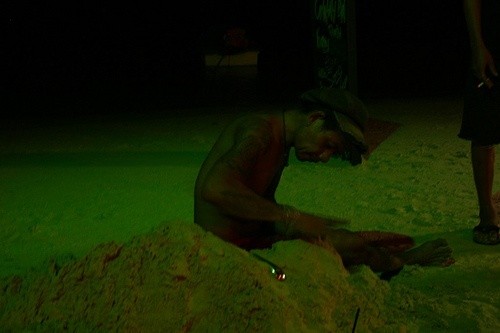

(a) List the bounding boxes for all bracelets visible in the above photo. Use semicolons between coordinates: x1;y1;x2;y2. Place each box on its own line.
274;202;303;238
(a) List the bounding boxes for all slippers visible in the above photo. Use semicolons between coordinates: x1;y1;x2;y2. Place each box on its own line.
472;224;500;245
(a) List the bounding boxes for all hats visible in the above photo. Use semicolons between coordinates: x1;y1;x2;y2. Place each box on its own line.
300;87;370;148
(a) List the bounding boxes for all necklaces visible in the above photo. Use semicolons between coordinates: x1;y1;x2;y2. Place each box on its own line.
279;107;293;170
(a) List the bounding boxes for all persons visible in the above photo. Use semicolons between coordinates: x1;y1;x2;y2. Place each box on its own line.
193;87;410;281
453;0;500;250
